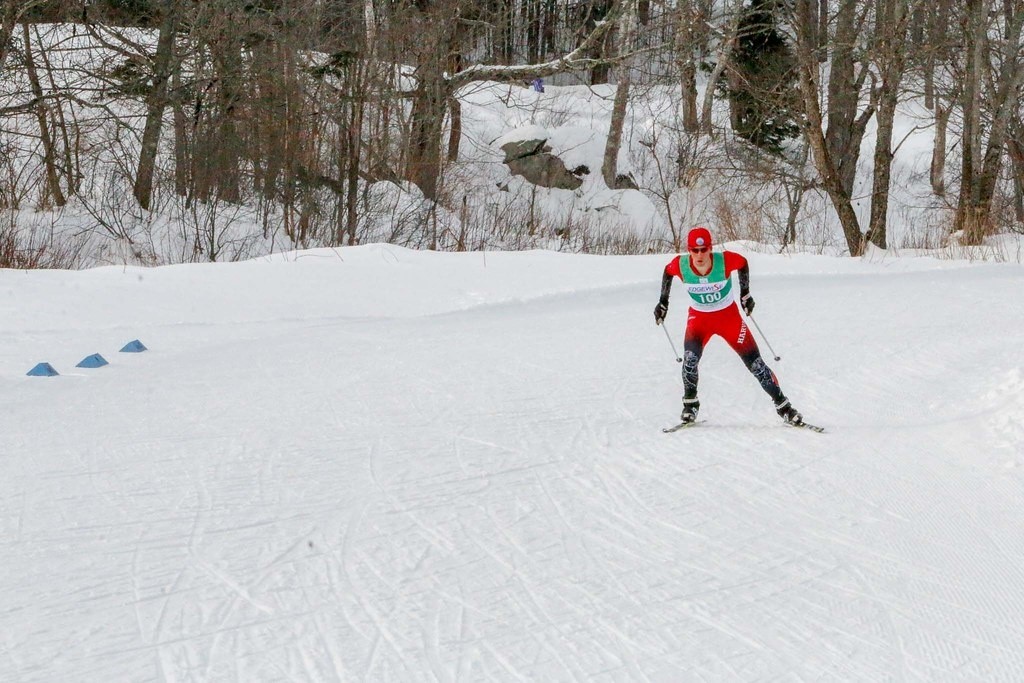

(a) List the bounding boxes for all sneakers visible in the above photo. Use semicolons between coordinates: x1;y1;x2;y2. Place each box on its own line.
775;398;803;425
681;397;700;422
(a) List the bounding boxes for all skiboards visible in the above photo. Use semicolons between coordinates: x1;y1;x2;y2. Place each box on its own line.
661;418;826;434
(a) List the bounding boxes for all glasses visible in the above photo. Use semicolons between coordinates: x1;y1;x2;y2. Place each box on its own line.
690;247;709;253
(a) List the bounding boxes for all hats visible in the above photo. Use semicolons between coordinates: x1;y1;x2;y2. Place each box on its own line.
687;228;712;253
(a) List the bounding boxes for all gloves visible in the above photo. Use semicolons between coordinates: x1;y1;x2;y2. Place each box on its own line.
654;298;668;325
740;288;755;316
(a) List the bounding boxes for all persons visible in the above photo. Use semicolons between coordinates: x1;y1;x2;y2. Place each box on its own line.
653;228;803;424
